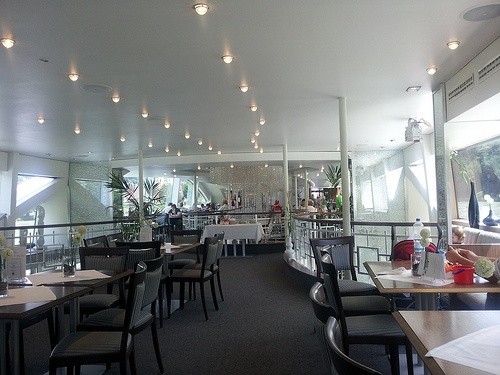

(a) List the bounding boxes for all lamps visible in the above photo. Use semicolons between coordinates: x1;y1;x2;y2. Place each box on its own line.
448;40;459;50
1;4;266;157
427;68;437;75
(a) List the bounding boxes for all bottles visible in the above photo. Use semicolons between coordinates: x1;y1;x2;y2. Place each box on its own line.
413;218;425;255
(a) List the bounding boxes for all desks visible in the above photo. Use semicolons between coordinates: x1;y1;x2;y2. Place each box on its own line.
142;242;201;316
363;261;500;375
391;310;500;375
204;222;262;259
0;284;88;375
9;267;135;375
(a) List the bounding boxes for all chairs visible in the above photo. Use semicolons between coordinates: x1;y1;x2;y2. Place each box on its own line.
263;208;338;239
49;214;224;375
308;235;413;375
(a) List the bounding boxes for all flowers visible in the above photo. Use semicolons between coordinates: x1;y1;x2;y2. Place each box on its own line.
449;144;493;211
419;228;431;251
68;226;86;266
0;234;13;269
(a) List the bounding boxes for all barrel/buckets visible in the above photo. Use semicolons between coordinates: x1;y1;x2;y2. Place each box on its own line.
451;265;474;284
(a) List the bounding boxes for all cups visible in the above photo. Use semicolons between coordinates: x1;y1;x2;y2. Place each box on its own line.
410;253;422;277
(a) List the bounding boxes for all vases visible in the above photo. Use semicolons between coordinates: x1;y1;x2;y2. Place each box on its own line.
62;258;75;277
417;250;427;274
0;269;10;298
468;180;498;229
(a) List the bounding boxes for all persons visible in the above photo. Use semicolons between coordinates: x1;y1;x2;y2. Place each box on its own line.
200;200;228;224
445;227;500;283
161;202;187;231
301;199;317;212
274;200;282;212
